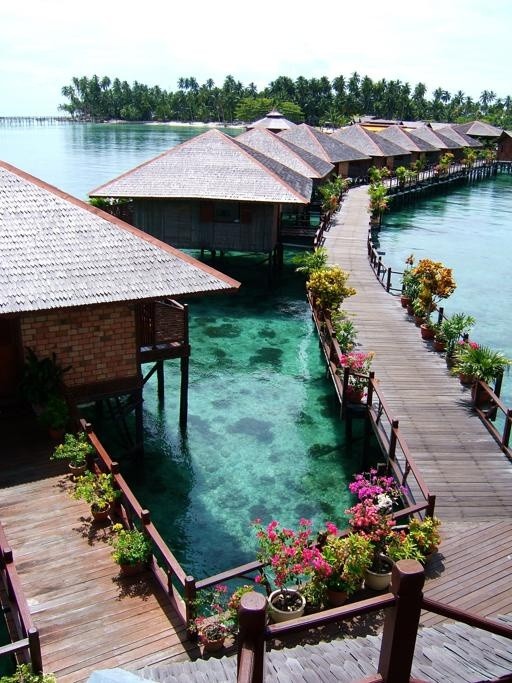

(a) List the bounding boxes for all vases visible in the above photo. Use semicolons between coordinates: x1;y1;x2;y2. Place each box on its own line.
366;553;394;591
267;587;307;621
200;638;226;654
419;547;438;571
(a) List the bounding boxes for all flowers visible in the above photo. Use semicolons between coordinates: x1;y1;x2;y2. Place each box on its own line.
407;515;444;553
346;500;401;570
186;582;257;642
250;515;332;608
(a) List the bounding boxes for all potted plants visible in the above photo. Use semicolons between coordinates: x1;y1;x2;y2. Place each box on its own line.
296;244;375;403
15;344;80;442
48;429;93;480
312;521;372;605
104;523;153;578
398;252;511;406
60;470;122;522
